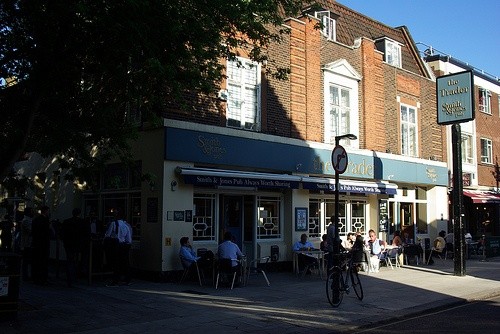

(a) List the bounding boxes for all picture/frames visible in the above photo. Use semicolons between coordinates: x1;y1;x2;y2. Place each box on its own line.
174;211;185;221
185;210;192;222
295;207;308;231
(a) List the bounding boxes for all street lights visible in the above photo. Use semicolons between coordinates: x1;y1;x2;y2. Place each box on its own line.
332;134;358;303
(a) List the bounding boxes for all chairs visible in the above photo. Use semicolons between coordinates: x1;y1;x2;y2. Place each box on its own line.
293;246;351;275
179;248;271;291
355;237;487;273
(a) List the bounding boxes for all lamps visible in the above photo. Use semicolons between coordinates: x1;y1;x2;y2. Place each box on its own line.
148;180;155;192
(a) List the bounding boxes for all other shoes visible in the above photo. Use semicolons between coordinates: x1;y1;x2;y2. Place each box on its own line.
105;283;119;288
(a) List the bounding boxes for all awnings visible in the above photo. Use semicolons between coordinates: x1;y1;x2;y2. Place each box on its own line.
293;174;398;194
174;167;301;189
463;191;500;203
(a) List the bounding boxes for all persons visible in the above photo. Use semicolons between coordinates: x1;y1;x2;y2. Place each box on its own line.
292;235;311;275
425;230;447;265
4;206;135;287
218;231;244;287
392;230;424;266
320;233;363;272
477;237;485;250
180;237;221;288
327;215;342;289
353;230;391;266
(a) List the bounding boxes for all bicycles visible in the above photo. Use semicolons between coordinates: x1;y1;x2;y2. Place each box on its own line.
325;251;369;308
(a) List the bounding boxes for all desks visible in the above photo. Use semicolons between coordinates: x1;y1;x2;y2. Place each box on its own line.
293;250;324;274
